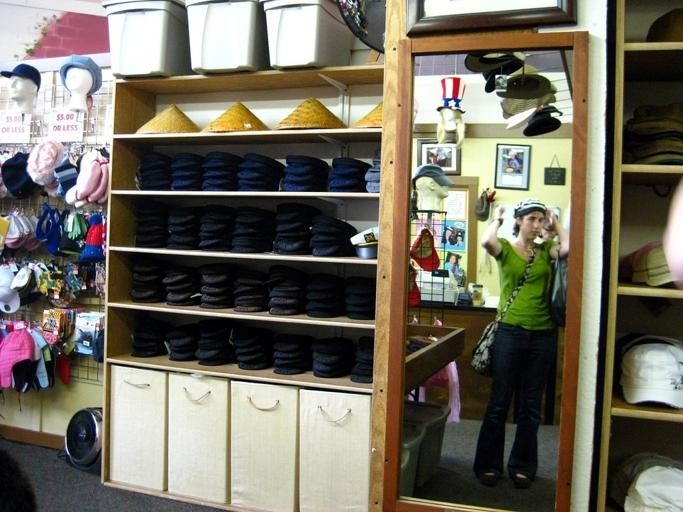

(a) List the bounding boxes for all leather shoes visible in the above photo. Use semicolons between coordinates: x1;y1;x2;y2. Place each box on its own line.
478;472;500;487
513;473;532;488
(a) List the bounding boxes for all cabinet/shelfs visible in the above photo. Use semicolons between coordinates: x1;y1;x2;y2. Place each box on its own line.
99;62;384;512
594;0;683;512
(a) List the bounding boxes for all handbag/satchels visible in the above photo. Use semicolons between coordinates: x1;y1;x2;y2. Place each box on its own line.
543;153;566;186
548;246;568;329
470;243;539;376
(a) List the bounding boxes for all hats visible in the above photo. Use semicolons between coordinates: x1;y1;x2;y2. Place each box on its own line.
198;263;233;309
622;104;682;165
201;151;241;190
351;335;374;383
202;102;272;131
436;78;466;114
1;322;56;393
60;54;102;96
309;215;358;257
306;272;345;318
167;207;201;250
620;332;683;411
233;271;268;312
513;200;547;218
273;202;322;255
647;8;683;42
506;108;537;130
233;327;272;370
84;156;109;209
131;201;169;248
475;190;490;222
624;466;683;512
131;320;168;357
2;152;35;200
26;141;64;185
163;265;201;306
500;93;556;119
262;265;304;315
281;155;331;191
237;152;286;191
507;65;538;80
65;154;101;205
631;240;680;287
231;206;274;253
0;265;20;314
345;276;376;320
412;164;455;191
409;228;440;272
135;104;199;134
36;203;71;258
169;153;204;191
1;64;41;88
364;149;381;192
350;101;383;127
464;50;517;73
273;334;309;375
409;264;420;306
312;337;354;378
199;204;232;251
482;64;525;93
330;157;372;192
191;319;233;366
276;98;347;129
130;260;164;303
606;452;682;507
495;75;551;99
166;323;199;362
75;150;108;208
524;112;561;136
80;214;105;262
136;152;173;190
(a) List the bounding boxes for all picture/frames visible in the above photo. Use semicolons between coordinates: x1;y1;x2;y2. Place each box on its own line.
495;143;532;191
416;138;461;176
400;0;578;35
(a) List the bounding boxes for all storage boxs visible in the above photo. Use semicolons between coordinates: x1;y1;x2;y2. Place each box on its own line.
102;0;184;77
258;0;353;69
183;0;261;72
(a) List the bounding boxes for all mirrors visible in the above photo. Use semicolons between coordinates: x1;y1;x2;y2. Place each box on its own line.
385;32;593;512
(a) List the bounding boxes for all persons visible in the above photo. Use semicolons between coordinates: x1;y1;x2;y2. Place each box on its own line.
444;252;459;275
443;225;465;248
0;64;41;113
413;164;454;211
473;200;565;487
427;151;437;162
507;150;522;174
436;76;466;147
61;54;102;109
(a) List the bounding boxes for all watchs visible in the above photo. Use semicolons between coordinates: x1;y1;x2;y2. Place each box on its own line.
492;218;503;224
